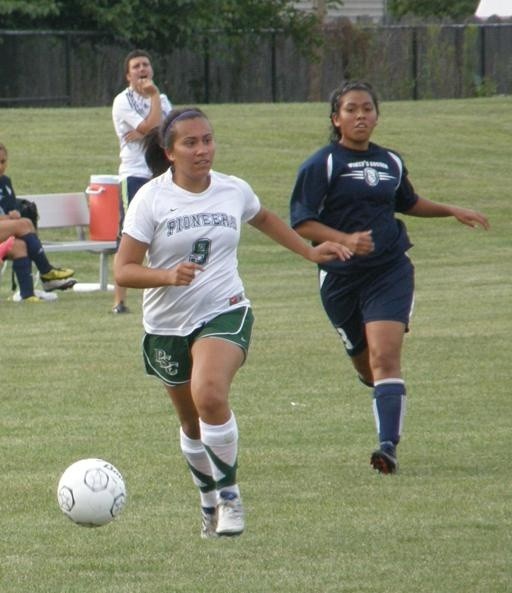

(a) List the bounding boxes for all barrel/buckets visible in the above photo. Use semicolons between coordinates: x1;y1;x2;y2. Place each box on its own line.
86;173;121;242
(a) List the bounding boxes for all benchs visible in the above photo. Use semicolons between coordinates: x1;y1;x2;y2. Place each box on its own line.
0;192;118;297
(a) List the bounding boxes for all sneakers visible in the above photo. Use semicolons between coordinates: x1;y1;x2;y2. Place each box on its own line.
21;295;49;303
201;507;217;539
40;266;74;282
42;278;77;292
370;446;398;474
216;485;243;536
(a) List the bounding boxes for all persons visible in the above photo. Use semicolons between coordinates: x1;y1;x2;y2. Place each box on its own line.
112;101;356;541
0;140;78;301
109;47;174;315
287;76;492;477
0;209;78;304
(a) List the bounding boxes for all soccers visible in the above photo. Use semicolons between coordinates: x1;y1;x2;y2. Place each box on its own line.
57;457;127;528
